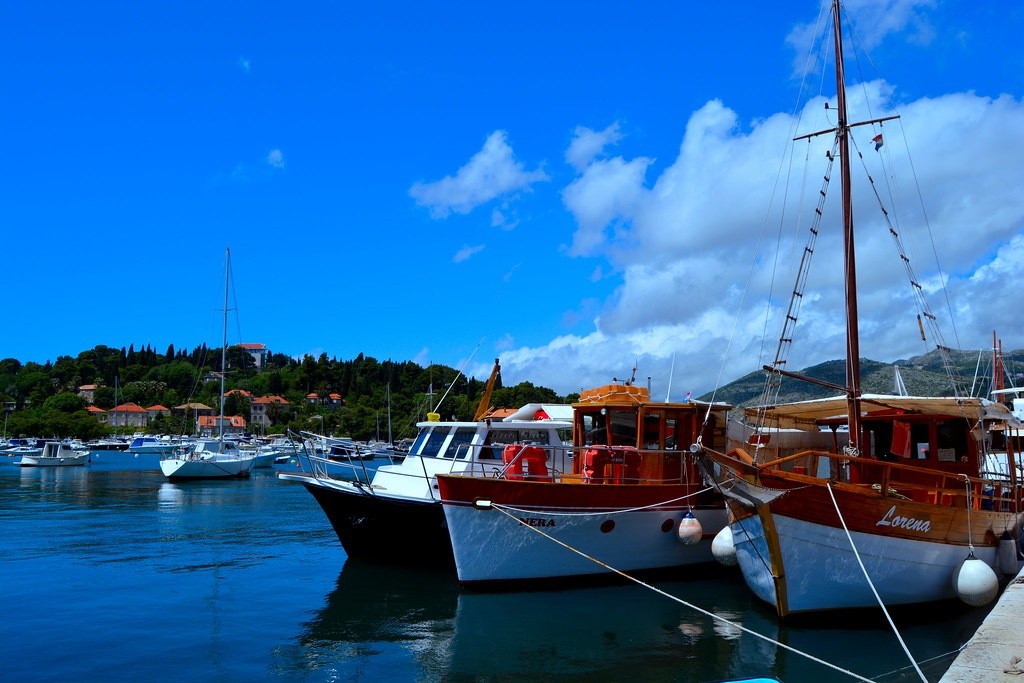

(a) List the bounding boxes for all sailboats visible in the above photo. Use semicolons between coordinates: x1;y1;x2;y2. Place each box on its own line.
1;2;1024;614
